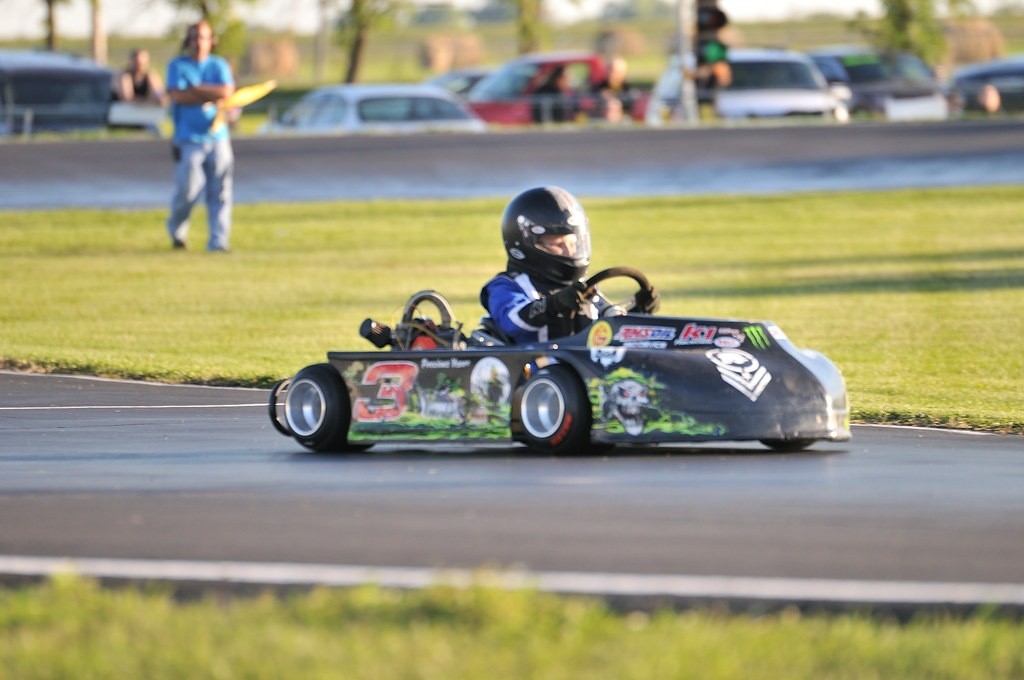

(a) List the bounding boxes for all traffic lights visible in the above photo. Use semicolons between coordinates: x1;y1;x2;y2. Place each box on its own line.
693;0;729;100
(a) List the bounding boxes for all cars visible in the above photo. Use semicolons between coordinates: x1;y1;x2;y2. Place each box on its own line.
433;49;658;129
948;55;1023;117
646;50;854;125
259;81;489;135
814;48;947;125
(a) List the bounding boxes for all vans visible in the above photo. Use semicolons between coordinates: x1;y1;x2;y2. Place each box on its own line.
0;51;118;137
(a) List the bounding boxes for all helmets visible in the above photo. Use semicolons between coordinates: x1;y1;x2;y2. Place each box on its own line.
500;185;591;282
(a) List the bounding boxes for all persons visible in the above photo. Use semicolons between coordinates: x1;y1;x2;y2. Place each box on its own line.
103;48;163;130
164;18;241;252
529;64;588;126
592;58;642;121
963;83;1007;118
478;185;659;344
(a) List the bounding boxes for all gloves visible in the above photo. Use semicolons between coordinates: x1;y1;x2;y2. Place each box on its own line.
547;281;588;312
634;285;661;315
